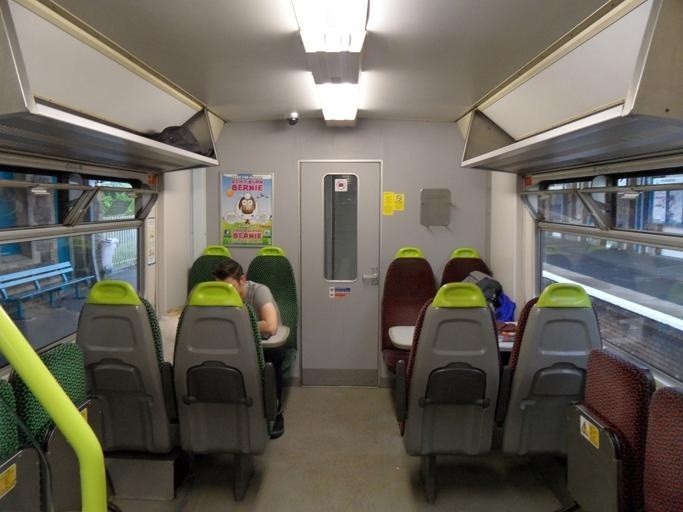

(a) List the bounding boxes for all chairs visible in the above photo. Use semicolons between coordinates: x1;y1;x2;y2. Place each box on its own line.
245;246;298;371
404;283;500;505
172;282;277;502
439;248;491;288
187;245;233;298
643;386;683;512
558;348;656;512
495;283;602;454
75;280;172;454
381;248;436;375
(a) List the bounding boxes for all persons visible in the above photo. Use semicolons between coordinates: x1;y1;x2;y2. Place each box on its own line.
214;258;285;440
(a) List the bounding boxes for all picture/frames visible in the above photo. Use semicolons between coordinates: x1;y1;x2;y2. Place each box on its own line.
219;172;275;248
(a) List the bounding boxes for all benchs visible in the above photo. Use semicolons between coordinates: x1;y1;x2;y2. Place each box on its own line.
0;261;96;319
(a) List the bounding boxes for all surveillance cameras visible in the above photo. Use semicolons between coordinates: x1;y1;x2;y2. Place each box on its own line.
287;112;299;125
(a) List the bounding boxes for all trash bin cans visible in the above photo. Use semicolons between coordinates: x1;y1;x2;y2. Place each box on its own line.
98;237;119;274
